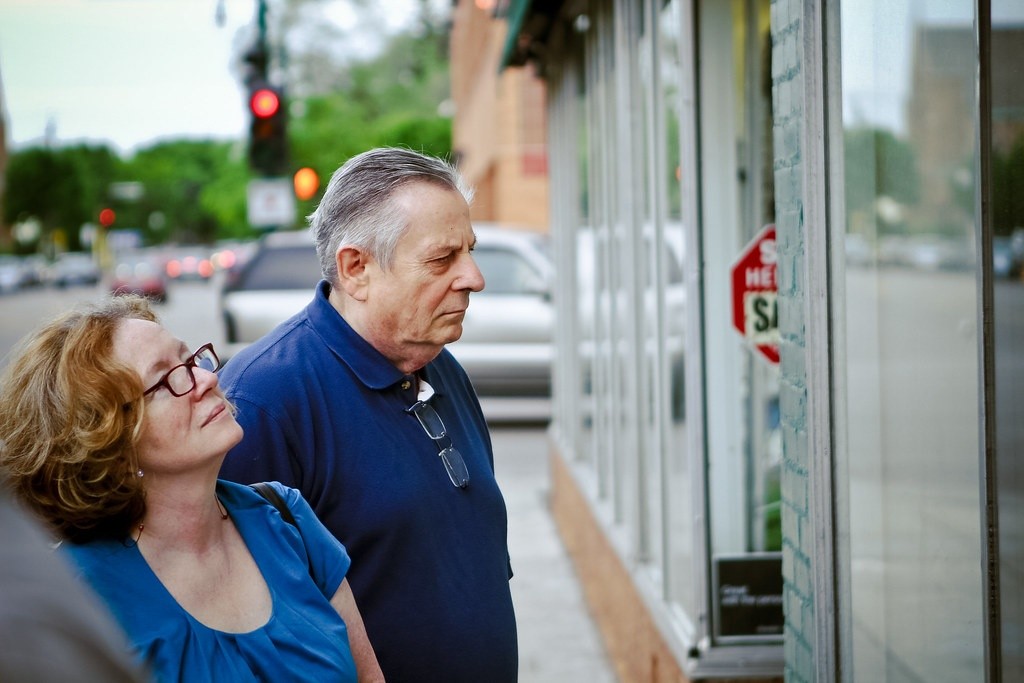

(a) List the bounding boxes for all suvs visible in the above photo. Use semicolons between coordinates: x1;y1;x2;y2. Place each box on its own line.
578;225;686;405
219;225;556;408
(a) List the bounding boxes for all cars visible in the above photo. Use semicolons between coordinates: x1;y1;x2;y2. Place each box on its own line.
112;261;169;304
844;230;1022;278
1;253;95;292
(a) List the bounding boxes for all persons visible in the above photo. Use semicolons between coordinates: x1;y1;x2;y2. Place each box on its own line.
216;146;519;683
0;293;388;683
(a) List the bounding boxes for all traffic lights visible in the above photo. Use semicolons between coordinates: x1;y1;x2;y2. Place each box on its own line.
248;85;287;175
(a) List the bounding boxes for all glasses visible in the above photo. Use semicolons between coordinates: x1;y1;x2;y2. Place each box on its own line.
413;400;470;489
121;341;221;409
